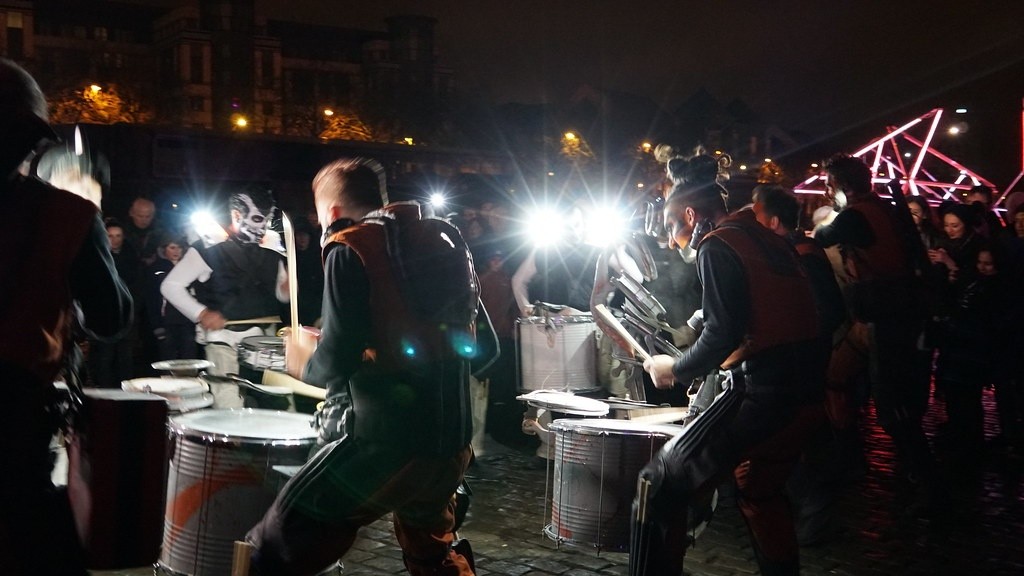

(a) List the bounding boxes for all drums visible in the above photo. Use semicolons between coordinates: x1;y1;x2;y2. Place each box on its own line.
155;405;343;576
237;331;322;413
57;386;169;573
121;376;217;461
546;409;690;553
515;313;602;395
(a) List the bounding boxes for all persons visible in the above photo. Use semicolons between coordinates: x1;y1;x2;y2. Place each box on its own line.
511;139;1023;576
0;59;520;576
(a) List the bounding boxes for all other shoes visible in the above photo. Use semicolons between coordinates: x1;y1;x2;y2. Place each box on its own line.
796;507;847;545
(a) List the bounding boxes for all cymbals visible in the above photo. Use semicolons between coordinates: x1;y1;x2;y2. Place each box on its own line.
149;358;217;374
597;394;659;407
516;390;609;417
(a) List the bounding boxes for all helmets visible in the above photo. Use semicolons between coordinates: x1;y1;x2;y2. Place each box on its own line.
0;57;62;143
